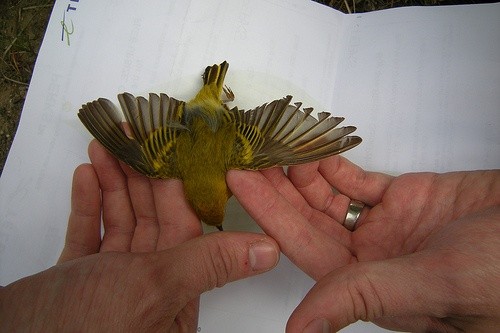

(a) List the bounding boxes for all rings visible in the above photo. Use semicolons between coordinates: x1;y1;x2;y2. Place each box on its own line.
344;197;365;230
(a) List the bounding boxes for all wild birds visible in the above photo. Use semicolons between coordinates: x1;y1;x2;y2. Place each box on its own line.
77;60;363;231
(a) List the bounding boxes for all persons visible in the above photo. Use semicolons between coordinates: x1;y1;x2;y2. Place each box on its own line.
0;120;500;333
